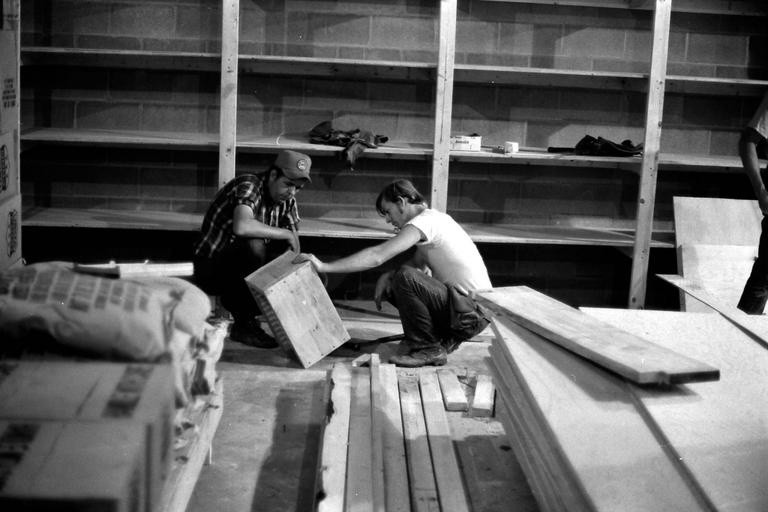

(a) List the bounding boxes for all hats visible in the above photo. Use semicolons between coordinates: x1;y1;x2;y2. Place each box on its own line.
271;148;315;184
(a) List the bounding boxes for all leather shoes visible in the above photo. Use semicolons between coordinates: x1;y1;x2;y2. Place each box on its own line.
387;341;449;368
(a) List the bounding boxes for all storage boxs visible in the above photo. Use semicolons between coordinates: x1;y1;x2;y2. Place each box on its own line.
0;418;152;511
0;361;177;511
249;250;348;369
1;0;27;271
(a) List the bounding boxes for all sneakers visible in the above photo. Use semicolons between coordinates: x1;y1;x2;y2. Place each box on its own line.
226;318;280;351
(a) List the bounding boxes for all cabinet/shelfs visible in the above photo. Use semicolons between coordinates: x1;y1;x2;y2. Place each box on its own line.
20;0;766;347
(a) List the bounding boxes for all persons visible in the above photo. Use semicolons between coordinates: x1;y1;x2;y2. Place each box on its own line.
292;175;495;368
737;93;767;318
186;150;314;349
